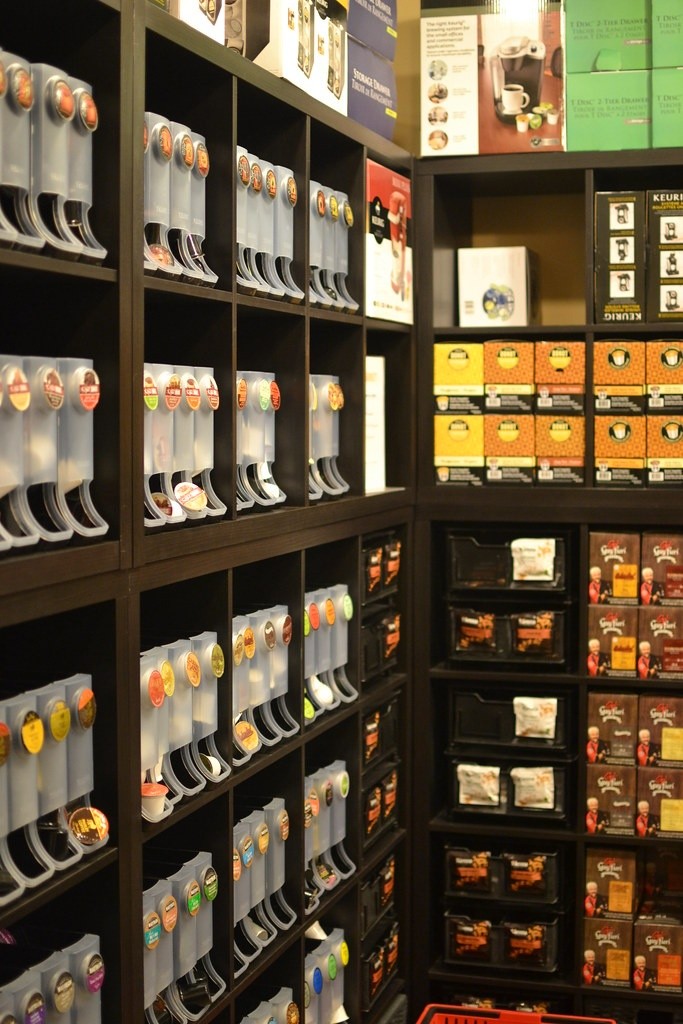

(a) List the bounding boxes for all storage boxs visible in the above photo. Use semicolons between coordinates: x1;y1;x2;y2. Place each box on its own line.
366;160;683;327
361;529;683;1023
147;0;683;158
365;336;683;493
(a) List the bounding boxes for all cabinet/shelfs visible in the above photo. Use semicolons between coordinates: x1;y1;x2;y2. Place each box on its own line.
133;0;417;568
415;147;683;1023
1;570;132;1024
0;0;133;595
133;506;414;1024
